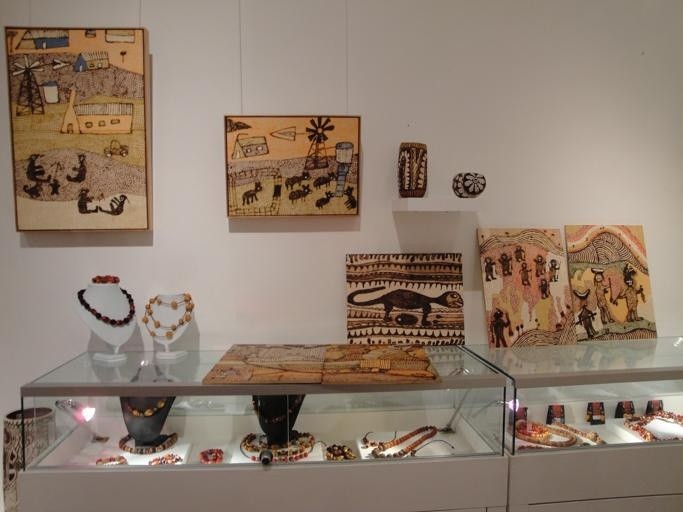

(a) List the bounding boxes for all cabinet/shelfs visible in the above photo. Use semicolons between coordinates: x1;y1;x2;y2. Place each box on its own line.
465;336;681;511
14;335;509;512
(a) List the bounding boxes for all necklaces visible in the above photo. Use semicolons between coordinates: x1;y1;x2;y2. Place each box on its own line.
510;410;683;450
77;275;194;339
252;395;302;424
126;397;168;417
